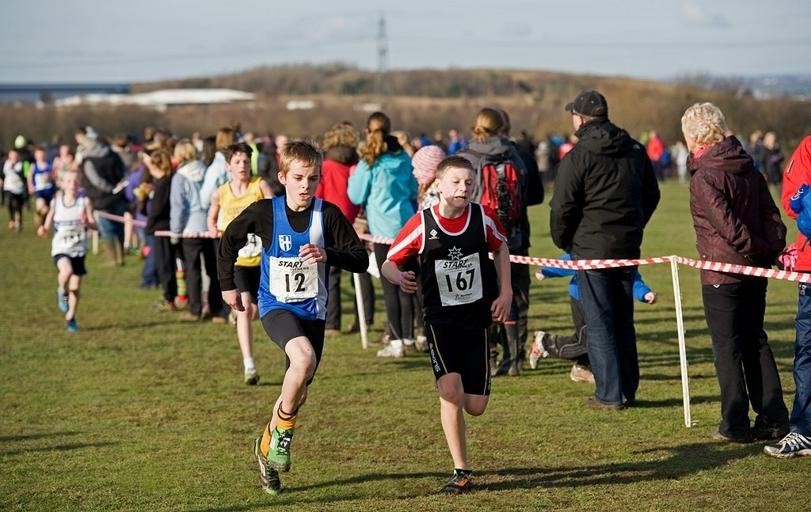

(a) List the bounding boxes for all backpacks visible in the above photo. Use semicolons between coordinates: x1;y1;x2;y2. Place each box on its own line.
463;142;521;236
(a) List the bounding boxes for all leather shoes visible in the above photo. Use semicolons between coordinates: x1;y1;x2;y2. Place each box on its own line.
587;396;624;411
710;428;754;442
622;393;636;406
751;422;790;440
178;311;200;322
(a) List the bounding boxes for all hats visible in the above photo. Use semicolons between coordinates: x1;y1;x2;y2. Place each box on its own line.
411;145;447;184
565;89;608;118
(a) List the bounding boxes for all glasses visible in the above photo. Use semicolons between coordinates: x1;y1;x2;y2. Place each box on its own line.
570;108;577;116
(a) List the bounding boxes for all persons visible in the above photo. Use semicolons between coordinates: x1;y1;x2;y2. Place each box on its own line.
26;145;54;217
682;103;789;440
199;126;238;212
409;146;445;211
73;125;96;157
549;89;662;409
208;145;275;384
143;149;181;309
390;128;430;158
173;139;222;323
316;121;375;335
114;151;151;252
126;127;173;151
457;109;532;377
39;161;100;331
432;129;466;153
193;132;213;164
499;109;542;372
347;112;420;357
50;144;72;172
747;128;786;183
382;156;512;494
216;141;370;493
243;131;289;194
532;128;580;172
5;149;28;232
76;137;127;264
530;251;656;383
644;130;690;183
764;131;811;456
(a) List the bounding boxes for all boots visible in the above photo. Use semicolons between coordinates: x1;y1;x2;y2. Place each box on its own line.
489;350;501;377
504;320;518;376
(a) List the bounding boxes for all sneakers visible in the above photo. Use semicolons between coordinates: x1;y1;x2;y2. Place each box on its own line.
401;338;418;354
377;339;406;358
380;334;391;344
174;296;188;311
267;424;294;473
415;335;429;352
65;314;78;333
8;221;14;229
155;300;176;313
343;324;371;335
570;363;596;383
56;287;69;313
441;469;473;494
252;434;282;496
243;358;260;385
324;327;343;339
763;431;811;457
529;330;550;370
14;223;21;233
